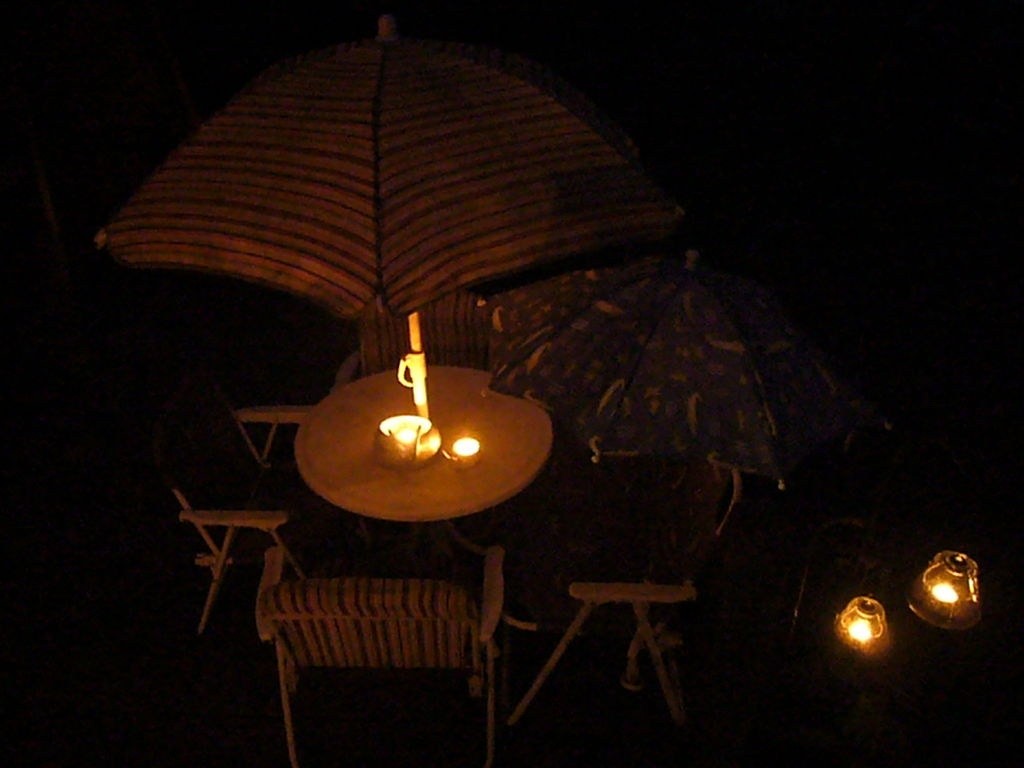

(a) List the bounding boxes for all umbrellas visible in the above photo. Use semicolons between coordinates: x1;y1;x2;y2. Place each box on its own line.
467;249;867;491
89;13;689;323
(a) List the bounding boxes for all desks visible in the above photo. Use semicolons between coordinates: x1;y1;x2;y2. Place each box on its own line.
292;365;553;522
779;514;933;737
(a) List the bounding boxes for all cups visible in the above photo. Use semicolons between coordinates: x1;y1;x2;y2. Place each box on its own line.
451;437;481;467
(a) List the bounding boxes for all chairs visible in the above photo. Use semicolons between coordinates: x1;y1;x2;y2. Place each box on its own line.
499;457;745;725
256;544;506;768
147;369;308;633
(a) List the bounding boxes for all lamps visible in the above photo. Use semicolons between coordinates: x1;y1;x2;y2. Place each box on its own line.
832;597;890;697
905;550;982;630
